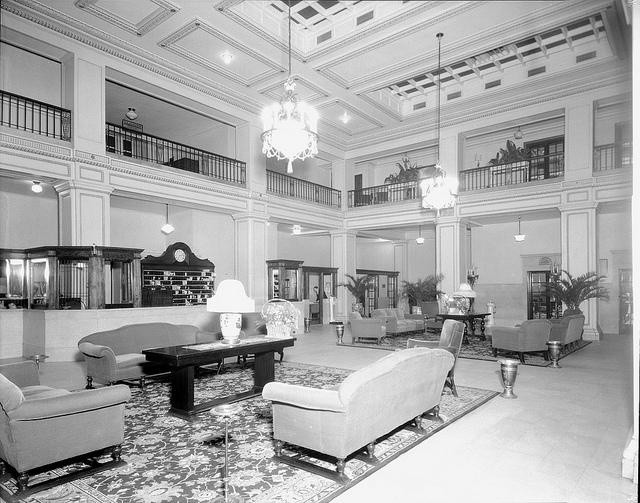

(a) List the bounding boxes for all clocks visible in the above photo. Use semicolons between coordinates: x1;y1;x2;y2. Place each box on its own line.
174;248;186;263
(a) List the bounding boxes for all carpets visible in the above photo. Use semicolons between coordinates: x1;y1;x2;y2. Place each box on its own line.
0;359;500;503
344;327;594;369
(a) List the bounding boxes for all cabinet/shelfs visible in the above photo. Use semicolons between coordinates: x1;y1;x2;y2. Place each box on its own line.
306;299;321;320
143;265;215;308
267;266;303;302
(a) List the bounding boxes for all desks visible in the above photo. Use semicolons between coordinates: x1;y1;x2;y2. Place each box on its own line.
136;331;301;421
434;307;492;344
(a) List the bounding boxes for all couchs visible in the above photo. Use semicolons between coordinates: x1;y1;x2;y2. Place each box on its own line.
0;352;134;503
347;302;430;345
76;322;203;391
258;339;455;483
485;312;587;367
402;317;466;399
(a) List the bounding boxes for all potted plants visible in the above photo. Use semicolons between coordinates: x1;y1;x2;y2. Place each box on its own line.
397;273;447;314
335;272;377;313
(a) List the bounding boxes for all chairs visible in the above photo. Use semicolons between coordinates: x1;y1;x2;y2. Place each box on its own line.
262;295;300;337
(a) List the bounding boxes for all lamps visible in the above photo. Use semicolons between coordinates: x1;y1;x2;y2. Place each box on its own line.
205;277;257;344
158;203;175;235
28;178;44;194
420;30;461;219
416;224;424;244
515;126;524;139
261;0;321;174
453;282;476;312
218;49;235;64
289;222;304;235
125;105;139;119
513;215;526;241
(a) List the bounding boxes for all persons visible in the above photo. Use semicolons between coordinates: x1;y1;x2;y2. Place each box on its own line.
313;285;327;301
